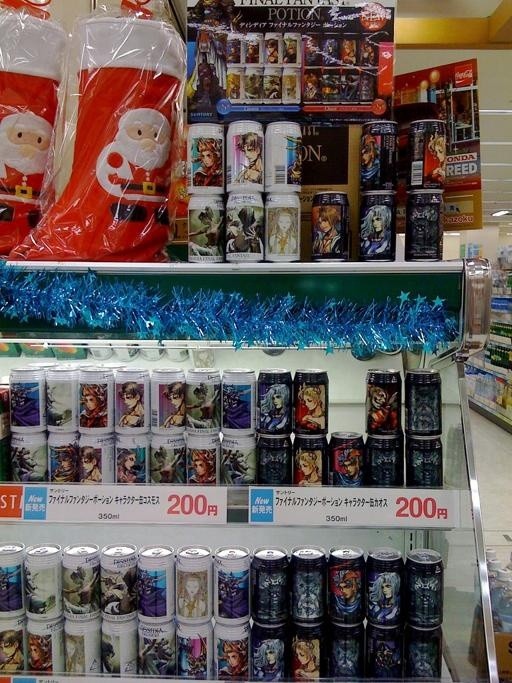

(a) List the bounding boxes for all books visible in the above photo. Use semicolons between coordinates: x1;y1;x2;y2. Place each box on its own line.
463;270;510;426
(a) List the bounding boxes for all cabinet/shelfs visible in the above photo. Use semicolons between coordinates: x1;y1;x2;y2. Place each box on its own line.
1;255;500;682
463;269;512;436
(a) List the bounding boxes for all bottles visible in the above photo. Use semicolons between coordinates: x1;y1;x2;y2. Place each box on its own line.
463;362;495;404
484;296;512;369
475;547;512;632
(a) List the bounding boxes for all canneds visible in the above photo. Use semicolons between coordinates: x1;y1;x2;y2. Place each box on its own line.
402;623;442;683
327;432;364;487
219;434;256;487
292;369;329;438
150;366;186;435
255;431;293;487
287;623;326;683
27;361;126;368
137;620;176;679
408;119;448;193
26;617;65;676
225;31;378;105
405;435;443;489
25;543;64;622
9;367;47;434
403;549;444;630
365;368;402;435
113;367;151;435
221;368;255;436
256;368;294;436
64;615;101;677
250;547;290;626
136;545;176;625
46;431;81;483
78;434;114;486
186;120;302;263
365;547;405;629
10;432;48;483
185;368;221;438
328;624;366;683
100;617;138;678
0;614;27;675
290;545;328;628
174;544;214;625
61;543;102;623
100;543;138;623
361;194;399;263
114;432;151;486
364;625;406;683
45;366;79;433
359;119;399;193
186;435;221;487
213;621;249;682
406;189;444;263
310;193;349;261
404;367;442;437
0;542;27;619
249;621;290;682
78;366;116;435
364;434;405;487
213;545;252;625
175;619;214;680
327;545;366;627
291;434;329;487
149;432;187;487
